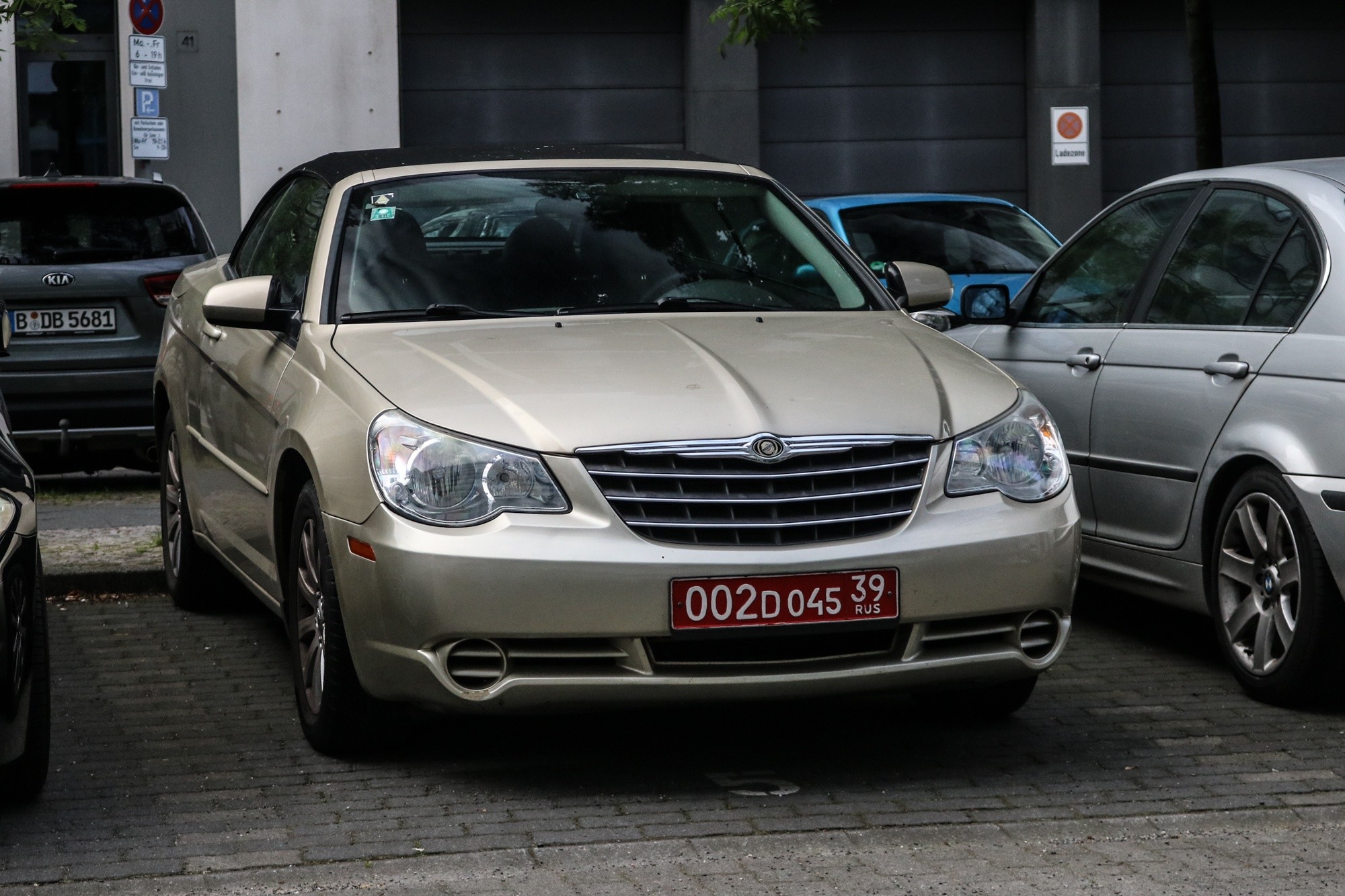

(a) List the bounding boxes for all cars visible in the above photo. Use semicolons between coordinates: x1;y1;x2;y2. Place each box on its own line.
726;192;1100;332
933;157;1345;701
0;418;53;799
150;149;1080;750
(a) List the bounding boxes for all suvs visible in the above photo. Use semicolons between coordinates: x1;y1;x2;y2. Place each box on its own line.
0;173;217;474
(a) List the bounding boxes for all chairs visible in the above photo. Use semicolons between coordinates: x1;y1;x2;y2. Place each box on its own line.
583;199;685;306
343;205;439;319
